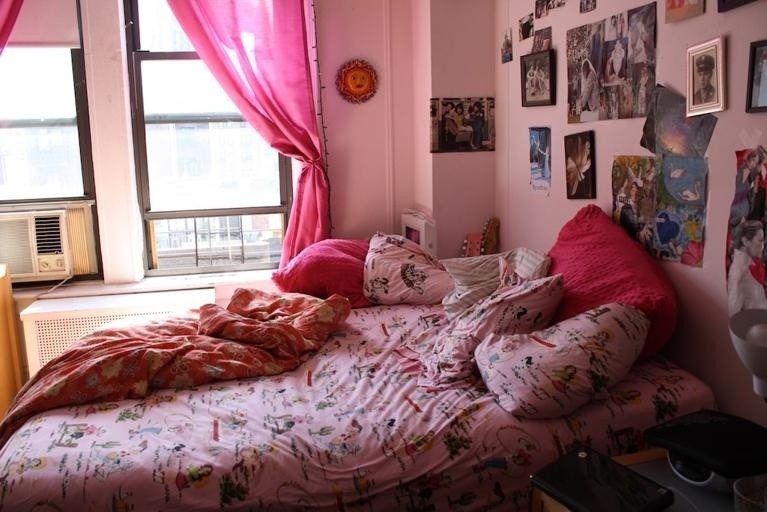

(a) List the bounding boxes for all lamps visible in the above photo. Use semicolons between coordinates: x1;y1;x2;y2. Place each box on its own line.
728;308;766;404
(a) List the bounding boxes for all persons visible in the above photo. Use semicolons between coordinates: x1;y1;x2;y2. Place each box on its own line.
530;134;540;162
502;1;593;63
440;102;484;149
567;137;591;196
694;56;716;104
754;54;766;106
527;61;549;94
727;146;767;312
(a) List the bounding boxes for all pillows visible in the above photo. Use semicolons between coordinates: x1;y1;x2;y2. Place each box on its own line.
271;238;377;310
547;205;676;352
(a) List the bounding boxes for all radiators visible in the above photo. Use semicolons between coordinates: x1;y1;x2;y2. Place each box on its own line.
21;287;218;382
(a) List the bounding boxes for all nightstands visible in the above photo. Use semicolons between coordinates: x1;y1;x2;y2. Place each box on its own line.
531;406;767;510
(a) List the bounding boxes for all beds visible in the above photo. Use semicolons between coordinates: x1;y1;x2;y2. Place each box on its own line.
0;251;721;512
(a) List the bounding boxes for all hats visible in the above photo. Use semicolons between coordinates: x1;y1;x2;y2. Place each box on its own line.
696;55;715;72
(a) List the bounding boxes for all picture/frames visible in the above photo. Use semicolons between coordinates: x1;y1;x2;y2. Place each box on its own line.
744;39;767;113
685;35;728;118
563;129;596;199
519;49;556;108
